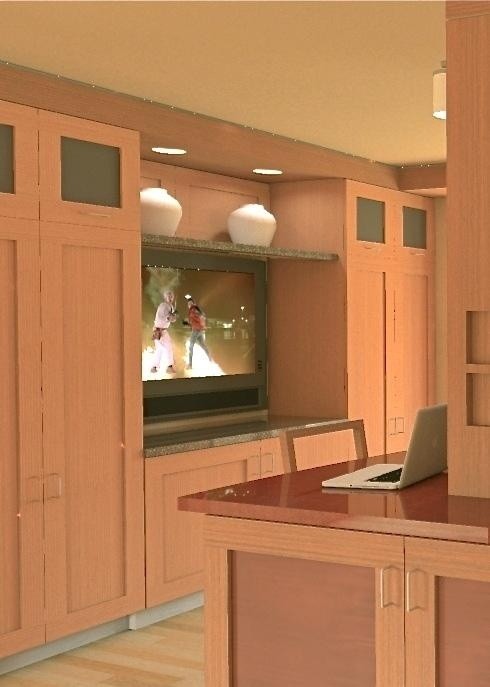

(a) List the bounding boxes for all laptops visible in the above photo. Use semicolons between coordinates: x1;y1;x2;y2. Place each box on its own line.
322;403;447;490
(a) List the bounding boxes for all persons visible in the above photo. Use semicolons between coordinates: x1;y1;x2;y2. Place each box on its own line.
149;291;178;373
182;299;213;370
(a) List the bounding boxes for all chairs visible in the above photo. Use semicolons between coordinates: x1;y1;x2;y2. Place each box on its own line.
277;418;368;472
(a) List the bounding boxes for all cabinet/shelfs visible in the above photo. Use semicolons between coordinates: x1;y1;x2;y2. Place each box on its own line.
2;217;145;663
145;427;349;608
268;257;433;459
1;98;144;231
201;513;490;687
271;177;437;257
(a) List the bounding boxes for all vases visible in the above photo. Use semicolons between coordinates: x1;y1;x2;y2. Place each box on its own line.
226;201;279;247
140;186;183;236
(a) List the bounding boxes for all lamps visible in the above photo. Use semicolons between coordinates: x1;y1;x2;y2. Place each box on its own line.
432;61;447;120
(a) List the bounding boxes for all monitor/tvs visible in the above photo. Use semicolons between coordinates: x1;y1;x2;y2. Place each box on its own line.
141;248;268;398
